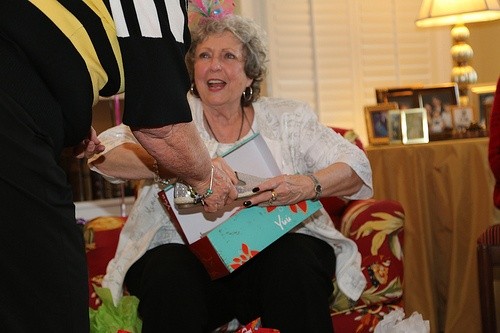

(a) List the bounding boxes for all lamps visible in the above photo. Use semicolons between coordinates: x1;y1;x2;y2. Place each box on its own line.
414;0;500;109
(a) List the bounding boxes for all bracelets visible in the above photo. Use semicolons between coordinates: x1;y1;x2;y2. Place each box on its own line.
189;164;213;205
152;158;162;182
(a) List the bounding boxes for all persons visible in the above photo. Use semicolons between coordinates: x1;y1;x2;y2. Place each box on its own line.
0;0;239;333
378;94;493;140
90;17;374;333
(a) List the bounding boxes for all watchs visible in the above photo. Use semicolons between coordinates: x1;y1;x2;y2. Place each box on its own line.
306;174;322;202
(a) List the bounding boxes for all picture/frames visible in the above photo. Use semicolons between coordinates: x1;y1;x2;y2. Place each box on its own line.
364;82;497;145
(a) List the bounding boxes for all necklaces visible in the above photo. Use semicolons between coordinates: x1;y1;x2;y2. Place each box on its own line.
204;105;247;143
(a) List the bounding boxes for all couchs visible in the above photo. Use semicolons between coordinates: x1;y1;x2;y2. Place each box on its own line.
86;127;407;333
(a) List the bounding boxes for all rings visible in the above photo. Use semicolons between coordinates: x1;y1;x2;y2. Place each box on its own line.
269;200;272;206
271;190;279;200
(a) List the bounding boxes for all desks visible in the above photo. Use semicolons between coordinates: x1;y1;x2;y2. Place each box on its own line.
360;137;500;333
